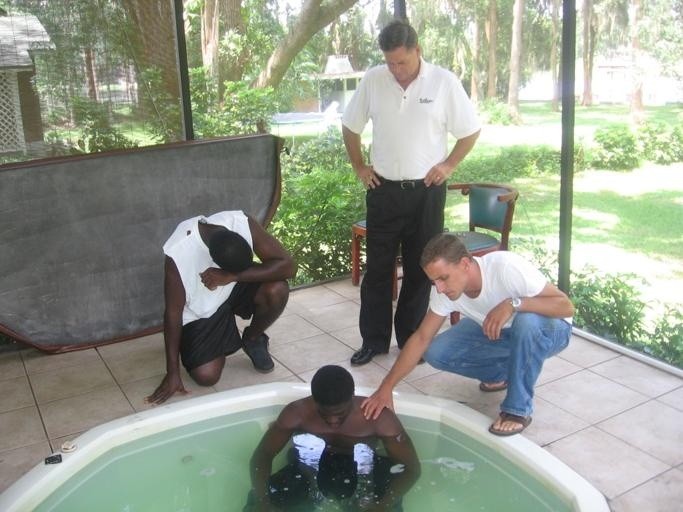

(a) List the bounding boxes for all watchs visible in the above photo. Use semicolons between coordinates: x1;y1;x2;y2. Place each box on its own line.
511;296;522;312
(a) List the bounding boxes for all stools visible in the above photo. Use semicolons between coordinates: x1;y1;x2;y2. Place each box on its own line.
351;219;404;300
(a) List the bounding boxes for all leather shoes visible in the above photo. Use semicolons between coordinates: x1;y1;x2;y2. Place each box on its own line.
350;345;382;366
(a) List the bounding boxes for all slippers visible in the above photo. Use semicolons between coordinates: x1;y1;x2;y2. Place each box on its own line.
489;413;532;436
480;379;508;392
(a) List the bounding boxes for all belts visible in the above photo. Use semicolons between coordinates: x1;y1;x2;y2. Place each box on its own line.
376;180;426;190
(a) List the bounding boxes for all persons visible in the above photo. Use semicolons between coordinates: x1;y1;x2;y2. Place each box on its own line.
243;365;421;512
361;234;574;436
340;22;483;367
144;210;297;407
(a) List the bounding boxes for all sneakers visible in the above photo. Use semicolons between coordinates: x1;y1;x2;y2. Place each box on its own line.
242;326;275;374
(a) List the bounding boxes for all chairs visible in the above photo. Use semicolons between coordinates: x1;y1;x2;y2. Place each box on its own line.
448;183;518;328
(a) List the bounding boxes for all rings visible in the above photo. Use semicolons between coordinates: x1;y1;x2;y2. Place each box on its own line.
437;176;440;180
